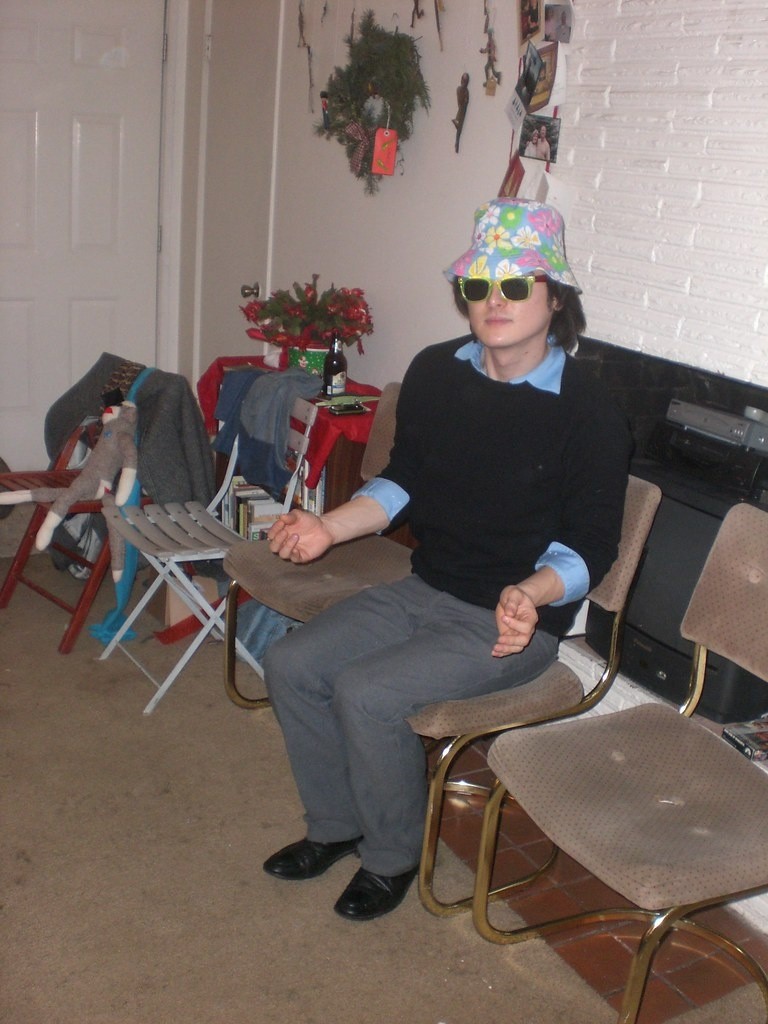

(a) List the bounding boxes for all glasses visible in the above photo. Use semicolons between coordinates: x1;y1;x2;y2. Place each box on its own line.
457;274;548;303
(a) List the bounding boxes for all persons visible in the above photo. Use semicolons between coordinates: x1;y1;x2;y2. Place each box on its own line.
521;0;538;36
263;197;631;921
524;126;550;160
545;7;570;43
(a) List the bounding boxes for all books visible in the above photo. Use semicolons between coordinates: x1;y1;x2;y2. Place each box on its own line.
222;460;323;540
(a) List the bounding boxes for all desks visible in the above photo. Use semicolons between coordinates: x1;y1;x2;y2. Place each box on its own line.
196;355;382;521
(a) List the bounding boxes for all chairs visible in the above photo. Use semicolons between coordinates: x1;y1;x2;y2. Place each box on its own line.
219;383;413;710
409;475;662;920
100;395;318;719
475;504;768;1024
0;350;197;655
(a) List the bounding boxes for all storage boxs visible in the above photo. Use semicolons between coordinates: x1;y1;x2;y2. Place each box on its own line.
146;563;219;628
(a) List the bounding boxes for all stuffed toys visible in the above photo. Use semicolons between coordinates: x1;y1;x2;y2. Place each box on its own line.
0;401;138;584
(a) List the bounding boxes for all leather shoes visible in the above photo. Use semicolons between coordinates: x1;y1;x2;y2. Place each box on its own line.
334;863;422;921
263;834;364;881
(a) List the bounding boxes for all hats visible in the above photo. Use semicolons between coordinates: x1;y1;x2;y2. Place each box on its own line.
443;197;582;294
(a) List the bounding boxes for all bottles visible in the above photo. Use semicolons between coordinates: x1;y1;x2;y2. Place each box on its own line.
323;329;346;397
